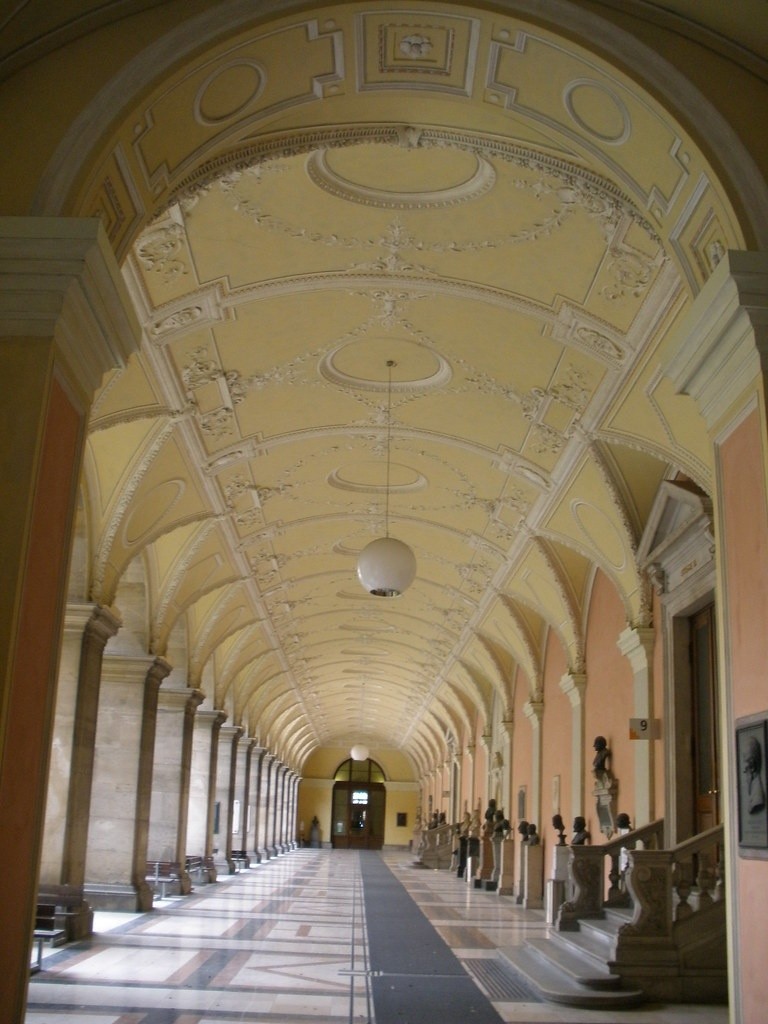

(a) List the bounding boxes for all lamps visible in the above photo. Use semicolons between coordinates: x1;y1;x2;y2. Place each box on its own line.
356;359;417;596
351;684;370;761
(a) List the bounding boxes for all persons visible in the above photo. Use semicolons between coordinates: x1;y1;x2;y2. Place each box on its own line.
571;816;591;844
552;815;565;831
592;736;611;781
459;798;512;838
517;820;539;845
428;813;446;829
616;813;635;849
